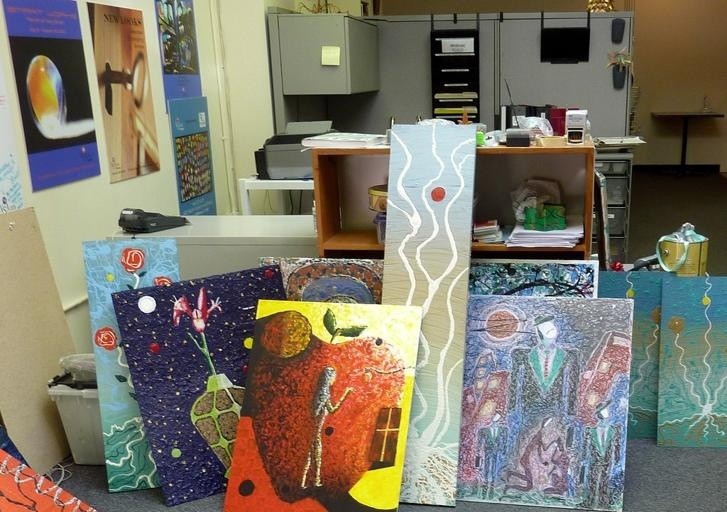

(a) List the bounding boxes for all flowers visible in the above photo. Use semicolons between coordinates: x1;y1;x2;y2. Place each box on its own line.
166;286;226;375
603;45;633;73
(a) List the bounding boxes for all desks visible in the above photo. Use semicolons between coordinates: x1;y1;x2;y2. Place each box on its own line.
650;112;724;163
237;177;315;215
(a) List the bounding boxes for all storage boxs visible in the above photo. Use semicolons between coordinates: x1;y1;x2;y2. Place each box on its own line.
373;213;386;245
48;384;109;468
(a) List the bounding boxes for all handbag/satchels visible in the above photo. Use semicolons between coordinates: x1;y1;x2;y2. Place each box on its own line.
512;189;550;223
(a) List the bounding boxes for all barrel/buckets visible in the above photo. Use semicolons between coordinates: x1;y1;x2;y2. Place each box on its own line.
657;233;708;278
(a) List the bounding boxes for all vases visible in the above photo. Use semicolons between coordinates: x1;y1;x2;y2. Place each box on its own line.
612;65;626;89
190;372;246;477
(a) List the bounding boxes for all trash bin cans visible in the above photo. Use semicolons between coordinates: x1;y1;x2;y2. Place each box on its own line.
47;354;104;465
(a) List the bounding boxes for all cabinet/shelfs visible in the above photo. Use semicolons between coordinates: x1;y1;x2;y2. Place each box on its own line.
591;148;634;264
102;211;320;280
312;134;596;260
276;11;379;97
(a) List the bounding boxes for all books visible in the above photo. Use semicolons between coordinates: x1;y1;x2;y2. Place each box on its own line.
594;134;648;147
301;132;384;147
472;217;582;250
